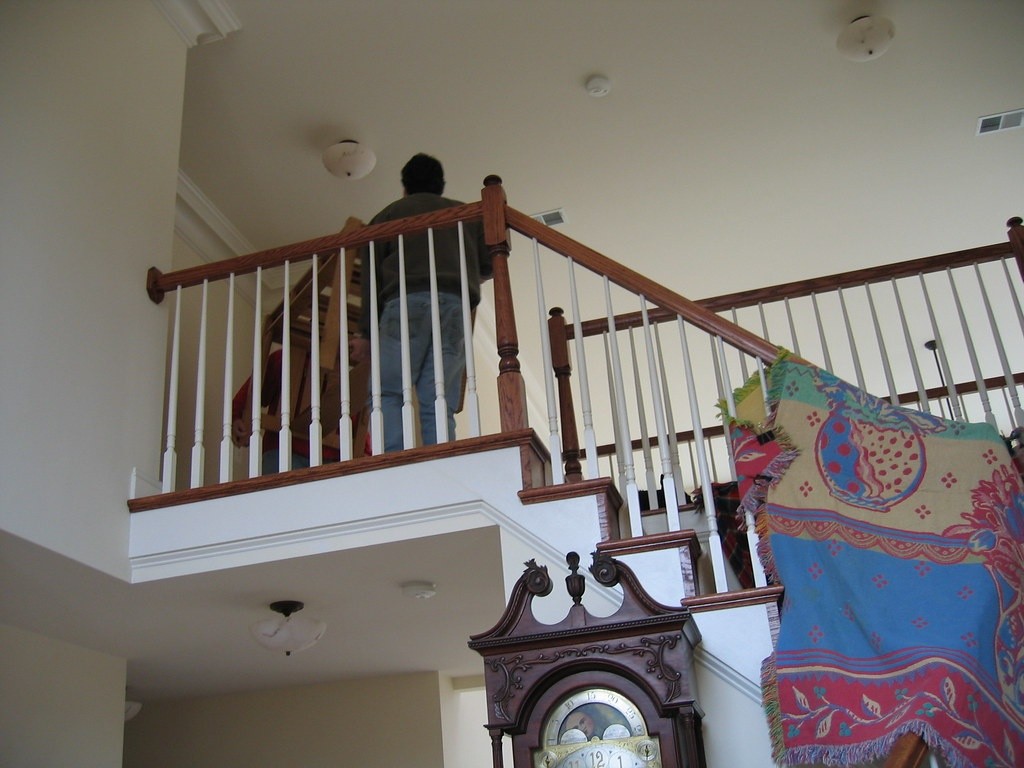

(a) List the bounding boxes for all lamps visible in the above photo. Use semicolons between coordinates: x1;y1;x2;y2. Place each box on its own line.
248;600;327;657
835;14;897;62
322;139;376;180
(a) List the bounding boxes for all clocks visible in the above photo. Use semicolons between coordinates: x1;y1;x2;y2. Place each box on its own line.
466;551;707;768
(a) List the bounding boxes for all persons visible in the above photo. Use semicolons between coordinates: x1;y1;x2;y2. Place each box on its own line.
356;153;493;454
231;350;374;477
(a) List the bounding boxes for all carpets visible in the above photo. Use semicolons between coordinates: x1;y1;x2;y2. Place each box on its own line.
715;347;1024;768
